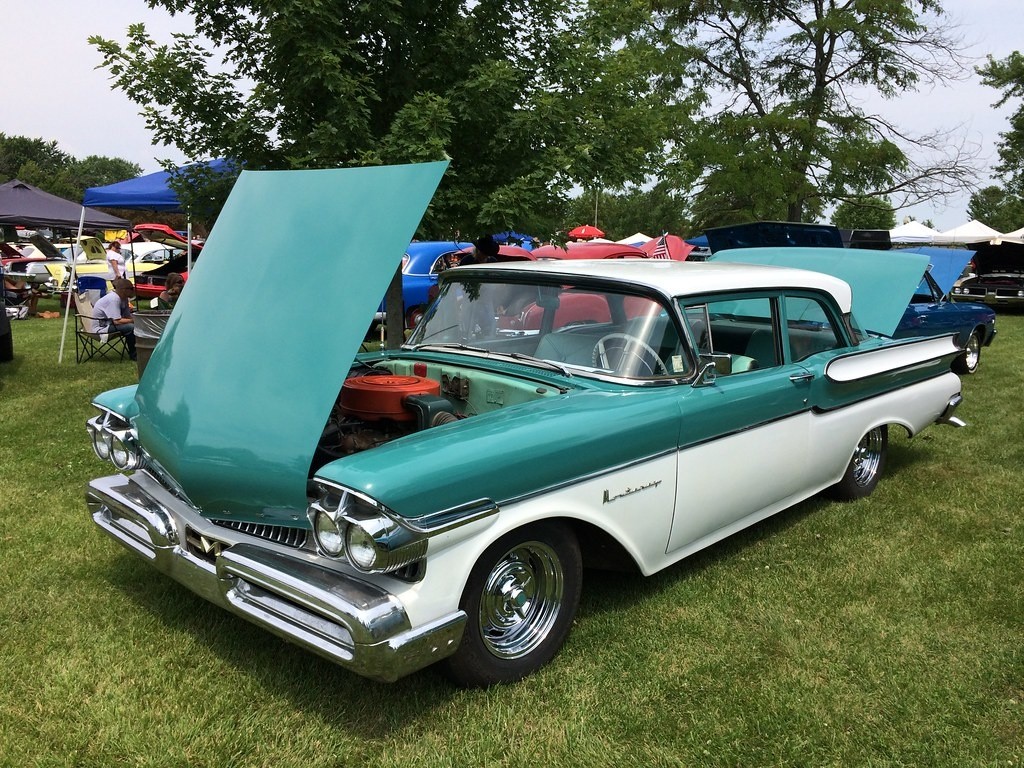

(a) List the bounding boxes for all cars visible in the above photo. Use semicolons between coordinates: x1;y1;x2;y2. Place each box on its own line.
372;241;475;332
84;158;963;688
452;235;701;332
893;246;997;375
132;222;203;297
0;234;205;292
949;242;1024;315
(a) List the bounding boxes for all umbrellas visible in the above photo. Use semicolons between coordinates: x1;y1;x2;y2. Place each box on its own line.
568;225;605;237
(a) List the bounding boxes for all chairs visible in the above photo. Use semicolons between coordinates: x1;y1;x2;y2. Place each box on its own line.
72;277;132;362
664;348;757;377
4;286;33;307
534;333;600;366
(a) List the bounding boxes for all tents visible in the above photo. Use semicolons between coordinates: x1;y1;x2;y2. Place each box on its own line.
0;178;131;231
83;156;251;212
889;219;1024;244
588;231;711;246
494;231;539;246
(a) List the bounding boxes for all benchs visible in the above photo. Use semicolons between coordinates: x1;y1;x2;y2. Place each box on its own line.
629;318;840;369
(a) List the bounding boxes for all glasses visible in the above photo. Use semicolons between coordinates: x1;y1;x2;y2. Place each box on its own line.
115;247;122;250
175;280;185;284
122;287;135;290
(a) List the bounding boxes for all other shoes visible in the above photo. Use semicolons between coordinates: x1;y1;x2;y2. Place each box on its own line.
27;312;37;317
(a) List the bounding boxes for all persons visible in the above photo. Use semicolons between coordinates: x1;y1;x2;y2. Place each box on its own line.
90;279;138;362
106;241;128;289
159;272;185;308
0;254;53;299
